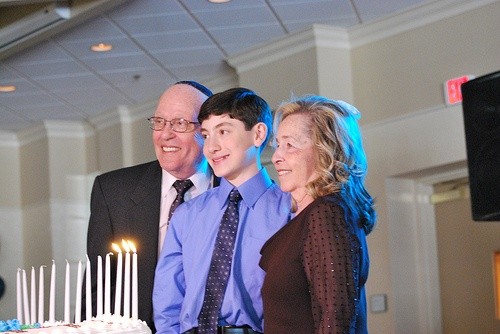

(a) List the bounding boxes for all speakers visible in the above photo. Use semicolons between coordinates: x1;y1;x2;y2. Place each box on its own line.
460;70;500;222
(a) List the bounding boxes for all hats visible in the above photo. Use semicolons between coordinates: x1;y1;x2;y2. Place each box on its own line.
175;81;214;98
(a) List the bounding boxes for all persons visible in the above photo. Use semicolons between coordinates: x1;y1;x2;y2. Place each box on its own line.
152;87;295;334
73;80;220;334
0;276;6;301
257;95;378;334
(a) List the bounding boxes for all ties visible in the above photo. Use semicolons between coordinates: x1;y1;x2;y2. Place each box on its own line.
197;190;243;334
165;178;195;232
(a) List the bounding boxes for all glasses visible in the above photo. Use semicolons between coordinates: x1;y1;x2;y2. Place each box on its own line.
147;116;201;134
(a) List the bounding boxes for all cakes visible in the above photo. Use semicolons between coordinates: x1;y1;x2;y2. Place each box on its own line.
0;313;152;334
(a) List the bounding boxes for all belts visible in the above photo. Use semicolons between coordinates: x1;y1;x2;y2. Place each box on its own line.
182;325;259;334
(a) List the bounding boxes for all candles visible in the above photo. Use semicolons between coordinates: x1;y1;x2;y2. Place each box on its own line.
15;267;22;326
122;239;130;318
131;242;139;319
65;259;71;323
38;264;47;324
30;265;36;323
97;254;102;315
85;252;92;319
48;258;55;322
113;242;122;317
22;269;29;324
104;253;113;315
74;259;82;323
